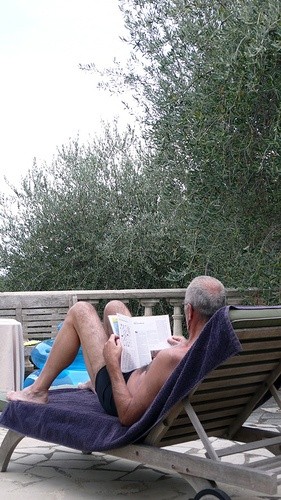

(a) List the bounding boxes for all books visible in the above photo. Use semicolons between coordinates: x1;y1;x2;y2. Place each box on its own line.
108;312;173;374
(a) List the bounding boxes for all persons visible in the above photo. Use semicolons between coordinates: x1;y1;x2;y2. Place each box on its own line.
8;274;229;426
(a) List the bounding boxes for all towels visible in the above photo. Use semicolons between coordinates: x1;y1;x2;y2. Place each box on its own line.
1;305;281;453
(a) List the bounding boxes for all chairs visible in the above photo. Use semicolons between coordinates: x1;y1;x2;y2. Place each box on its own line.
0;306;281;500
